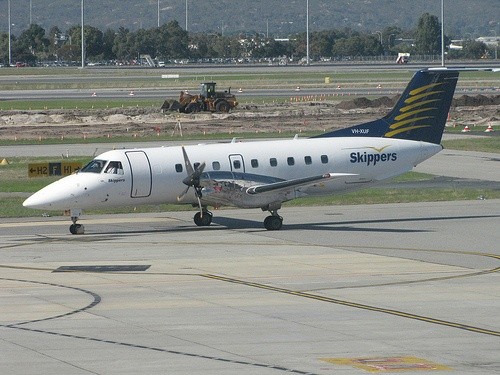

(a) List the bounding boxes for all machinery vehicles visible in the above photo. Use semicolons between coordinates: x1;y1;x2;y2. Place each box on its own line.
162;79;239;114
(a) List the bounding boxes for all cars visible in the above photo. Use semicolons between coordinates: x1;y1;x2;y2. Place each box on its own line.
1;57;167;67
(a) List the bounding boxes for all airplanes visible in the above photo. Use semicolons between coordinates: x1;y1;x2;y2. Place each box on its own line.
21;68;461;232
